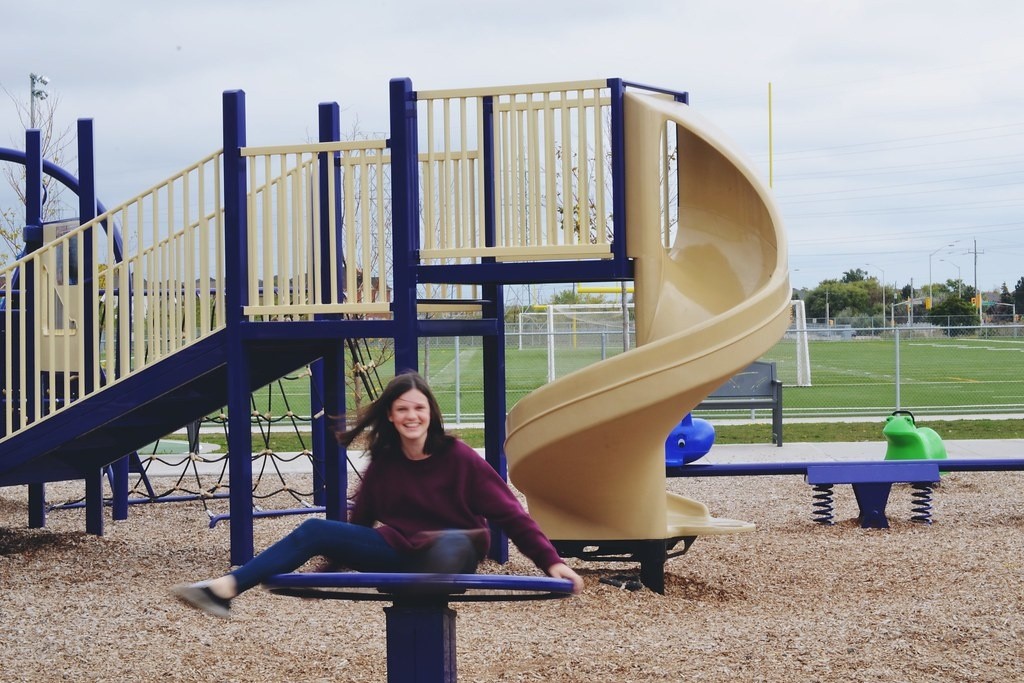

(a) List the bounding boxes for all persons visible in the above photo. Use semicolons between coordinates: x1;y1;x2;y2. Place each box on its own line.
165;372;585;618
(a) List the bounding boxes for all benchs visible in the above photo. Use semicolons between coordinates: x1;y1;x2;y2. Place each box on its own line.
694;360;783;447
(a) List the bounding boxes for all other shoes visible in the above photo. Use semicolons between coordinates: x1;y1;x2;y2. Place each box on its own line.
172;583;229;619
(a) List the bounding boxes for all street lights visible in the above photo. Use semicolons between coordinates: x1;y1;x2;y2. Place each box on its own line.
865;262;886;331
928;240;961;314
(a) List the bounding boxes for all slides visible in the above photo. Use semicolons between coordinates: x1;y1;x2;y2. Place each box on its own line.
504;89;793;543
